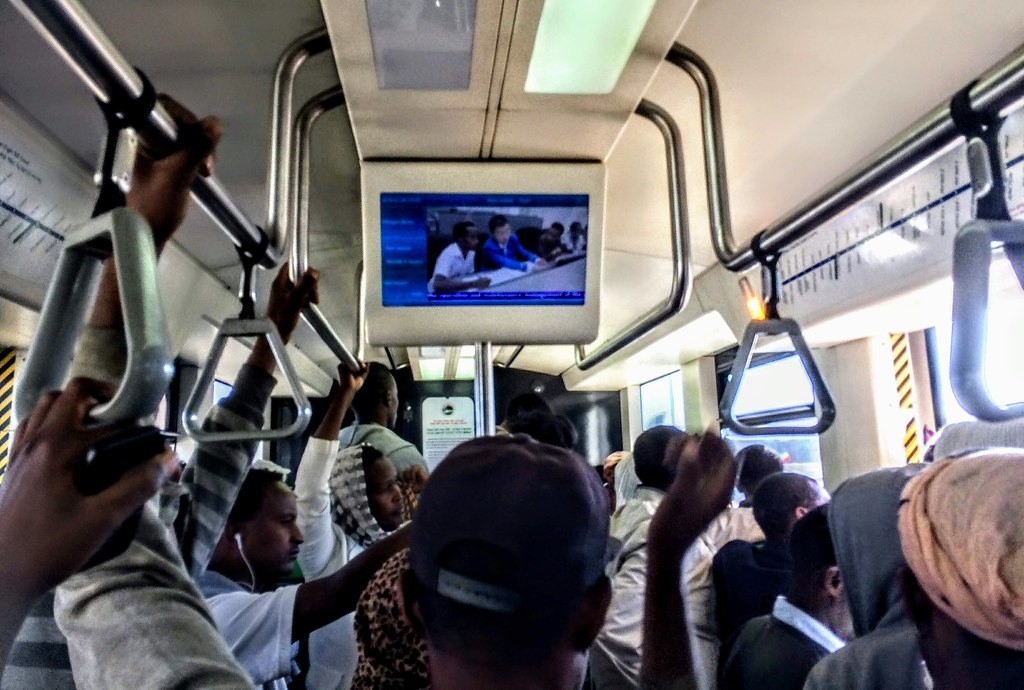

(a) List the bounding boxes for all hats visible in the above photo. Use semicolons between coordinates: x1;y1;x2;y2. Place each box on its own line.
411;430;609;613
900;448;1024;651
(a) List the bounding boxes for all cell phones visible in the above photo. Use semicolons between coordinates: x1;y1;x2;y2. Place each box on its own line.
72;426;161;569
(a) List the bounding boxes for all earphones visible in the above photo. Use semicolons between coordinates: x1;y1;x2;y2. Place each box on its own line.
234;532;243;551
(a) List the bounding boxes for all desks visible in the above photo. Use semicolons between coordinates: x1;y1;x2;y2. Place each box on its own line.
427;250;586;292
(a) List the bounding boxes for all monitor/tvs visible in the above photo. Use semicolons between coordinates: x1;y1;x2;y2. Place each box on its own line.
360;160;607;345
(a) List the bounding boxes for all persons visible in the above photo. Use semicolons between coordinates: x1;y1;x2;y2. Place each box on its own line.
47;93;613;688
0;371;181;690
733;440;787;548
331;360;428;560
514;406;579;455
478;212;549;276
561;220;586;253
1;260;323;690
490;390;554;453
801;441;1023;687
530;221;573;265
587;429;648;528
293;352;436;690
711;472;835;675
170;465;427;689
726;498;862;690
640;420;746;690
428;221;492;295
920;415;1024;492
801;460;952;690
596;423;721;690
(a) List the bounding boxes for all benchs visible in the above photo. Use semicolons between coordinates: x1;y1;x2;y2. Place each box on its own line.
437;210;543;248
